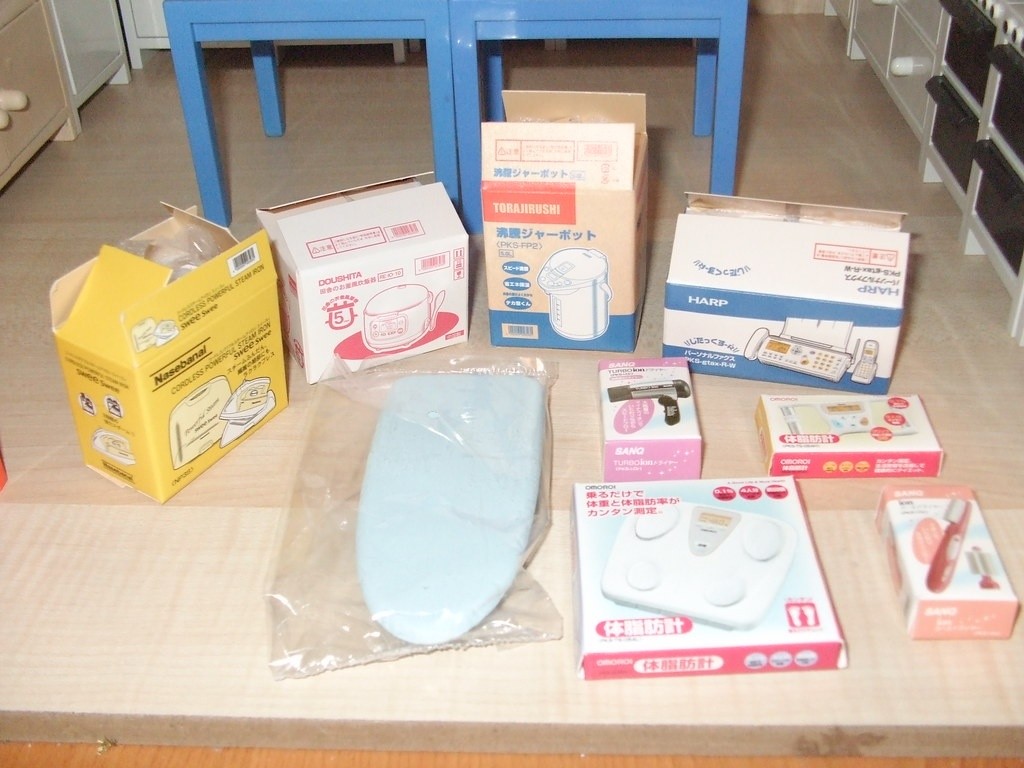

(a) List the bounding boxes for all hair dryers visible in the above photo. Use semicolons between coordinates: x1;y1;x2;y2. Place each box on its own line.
607;380;691;427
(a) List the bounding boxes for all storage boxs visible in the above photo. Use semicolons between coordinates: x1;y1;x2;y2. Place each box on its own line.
480;90;649;356
876;485;1018;639
755;395;943;479
662;192;910;395
571;359;847;680
256;170;468;385
50;200;289;505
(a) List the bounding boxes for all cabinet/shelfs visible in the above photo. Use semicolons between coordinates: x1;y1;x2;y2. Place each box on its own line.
824;0;1024;346
0;0;170;188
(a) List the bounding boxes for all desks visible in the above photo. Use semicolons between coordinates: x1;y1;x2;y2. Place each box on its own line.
162;0;749;234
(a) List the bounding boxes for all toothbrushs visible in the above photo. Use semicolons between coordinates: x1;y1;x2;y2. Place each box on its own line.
927;499;971;594
